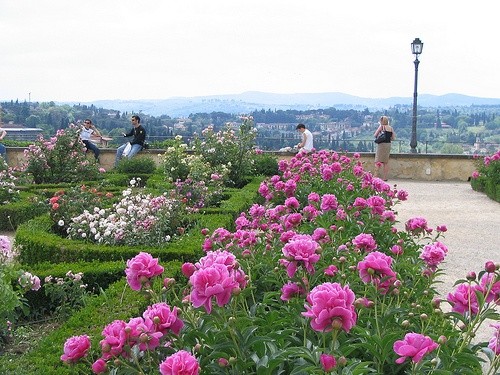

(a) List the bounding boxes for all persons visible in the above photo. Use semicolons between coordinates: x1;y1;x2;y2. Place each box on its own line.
373;114;395;181
295;123;313;154
0;127;7;162
79;119;101;159
111;116;146;170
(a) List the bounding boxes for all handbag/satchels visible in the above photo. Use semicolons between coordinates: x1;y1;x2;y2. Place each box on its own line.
122;143;131;157
374;125;386;143
143;140;149;148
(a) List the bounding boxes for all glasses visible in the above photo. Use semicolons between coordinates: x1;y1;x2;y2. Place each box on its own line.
85;122;89;124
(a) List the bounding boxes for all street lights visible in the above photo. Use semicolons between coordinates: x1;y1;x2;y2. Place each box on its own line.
410;37;423;154
